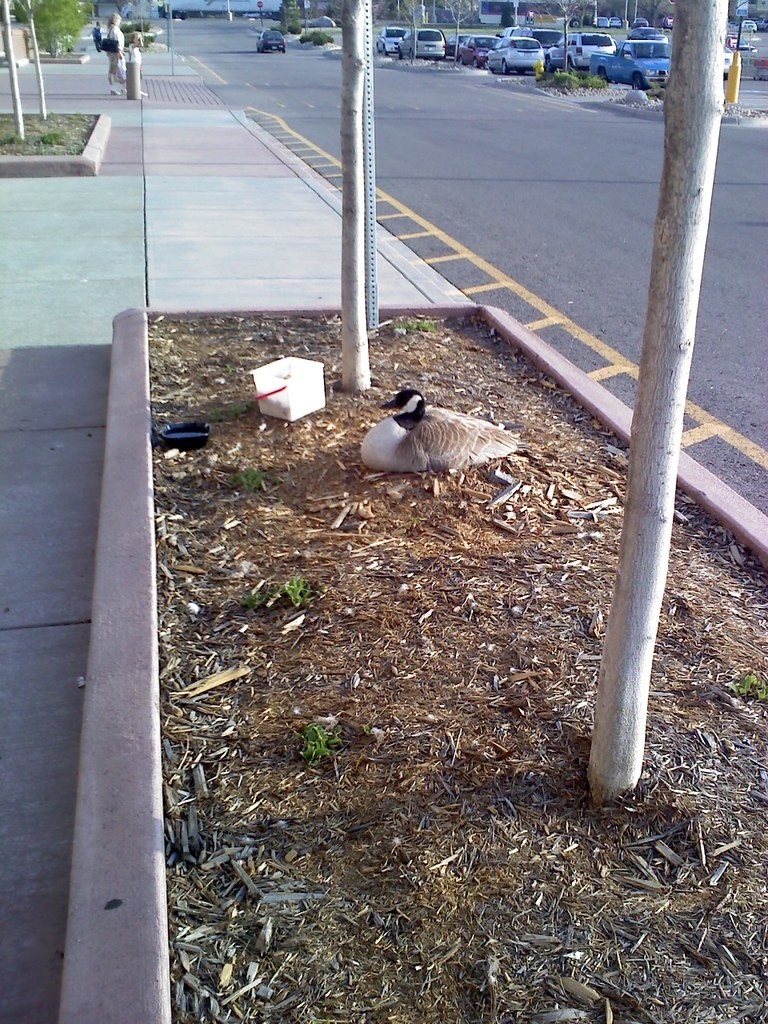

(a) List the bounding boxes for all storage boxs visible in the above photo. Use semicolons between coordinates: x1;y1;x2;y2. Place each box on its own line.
249;356;327;422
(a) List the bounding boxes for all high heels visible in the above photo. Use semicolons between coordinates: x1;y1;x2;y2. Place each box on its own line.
120;88;129;93
111;89;121;96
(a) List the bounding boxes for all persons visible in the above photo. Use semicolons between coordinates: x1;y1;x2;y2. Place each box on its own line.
107;12;127;96
129;30;144;79
92;21;106;52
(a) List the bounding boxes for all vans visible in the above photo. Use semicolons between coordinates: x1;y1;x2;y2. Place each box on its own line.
395;29;447;61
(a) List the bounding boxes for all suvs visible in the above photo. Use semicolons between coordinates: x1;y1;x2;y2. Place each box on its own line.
544;31;618;70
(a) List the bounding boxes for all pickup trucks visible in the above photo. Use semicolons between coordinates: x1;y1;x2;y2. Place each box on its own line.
590;39;674;91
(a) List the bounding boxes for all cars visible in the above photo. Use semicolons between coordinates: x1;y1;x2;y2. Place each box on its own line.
631;17;649;28
298;16;336;28
609;15;622;29
723;15;768;83
242;11;269;19
455;36;502;68
160;7;188;20
663;15;678;28
483;36;547;74
442;34;476;56
256;29;286;55
508;28;562;58
496;26;519;40
624;26;666;43
376;26;409;56
596;16;609;28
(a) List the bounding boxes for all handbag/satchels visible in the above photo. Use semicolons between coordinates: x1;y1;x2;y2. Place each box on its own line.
116;53;129;80
101;38;118;51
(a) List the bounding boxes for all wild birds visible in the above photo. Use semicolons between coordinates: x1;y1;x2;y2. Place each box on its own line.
359;389;518;473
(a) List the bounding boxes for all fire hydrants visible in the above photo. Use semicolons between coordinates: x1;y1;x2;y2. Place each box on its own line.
531;58;545;81
